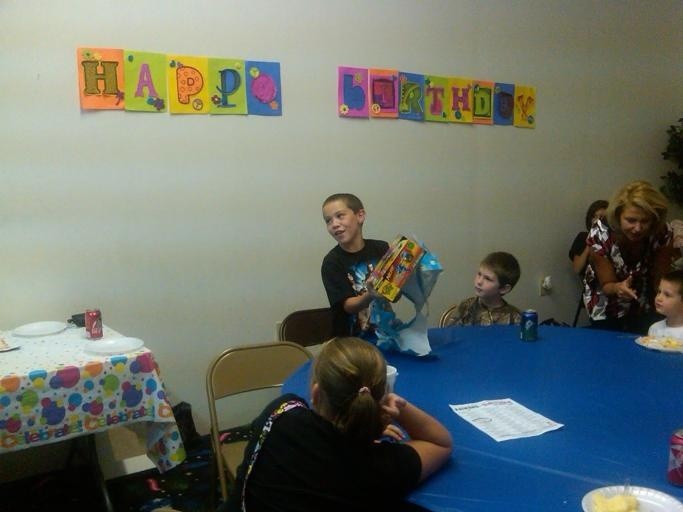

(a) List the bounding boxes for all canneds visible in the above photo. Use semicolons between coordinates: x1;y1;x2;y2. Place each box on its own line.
668;430;683;485
85;310;103;338
520;310;538;341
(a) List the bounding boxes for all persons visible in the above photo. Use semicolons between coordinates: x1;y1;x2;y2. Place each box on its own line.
647;270;683;342
224;337;455;512
568;199;609;276
317;191;402;340
580;179;683;337
442;251;527;327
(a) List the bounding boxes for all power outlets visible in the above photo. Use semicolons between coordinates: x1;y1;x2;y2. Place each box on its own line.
540;278;552;296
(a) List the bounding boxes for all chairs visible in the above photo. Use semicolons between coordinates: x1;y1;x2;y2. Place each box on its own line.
206;341;314;504
279;308;339;347
437;303;458;328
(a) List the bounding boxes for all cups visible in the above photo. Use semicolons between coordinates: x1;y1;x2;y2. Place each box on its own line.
384;365;397;395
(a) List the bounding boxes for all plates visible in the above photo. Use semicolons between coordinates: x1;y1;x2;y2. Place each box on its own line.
84;336;144;355
634;336;682;355
579;483;682;512
14;321;67;337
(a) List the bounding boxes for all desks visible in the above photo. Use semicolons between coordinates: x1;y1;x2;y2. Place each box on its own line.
0;324;153;512
